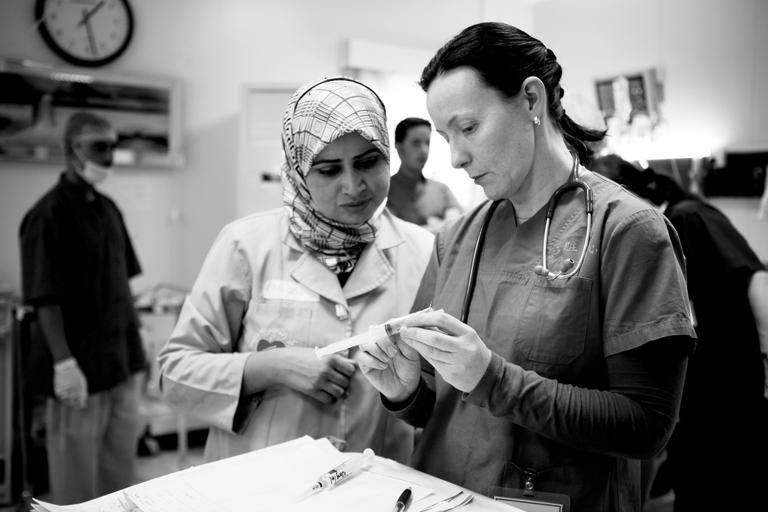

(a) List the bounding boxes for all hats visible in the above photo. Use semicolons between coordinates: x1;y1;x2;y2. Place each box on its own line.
66;113;117;143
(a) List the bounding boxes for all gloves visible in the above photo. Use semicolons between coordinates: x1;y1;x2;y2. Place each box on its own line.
54;358;89;410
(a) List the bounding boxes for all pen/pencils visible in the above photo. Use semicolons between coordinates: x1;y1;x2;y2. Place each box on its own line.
393;486;412;512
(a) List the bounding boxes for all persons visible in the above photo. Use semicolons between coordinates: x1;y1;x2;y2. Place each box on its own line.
589;151;767;512
355;20;698;512
385;117;465;235
18;109;148;504
156;72;432;467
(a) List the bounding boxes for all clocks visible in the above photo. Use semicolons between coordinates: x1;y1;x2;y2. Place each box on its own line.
34;1;135;68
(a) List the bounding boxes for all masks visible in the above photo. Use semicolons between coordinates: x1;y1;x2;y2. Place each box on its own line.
72;148;113;185
